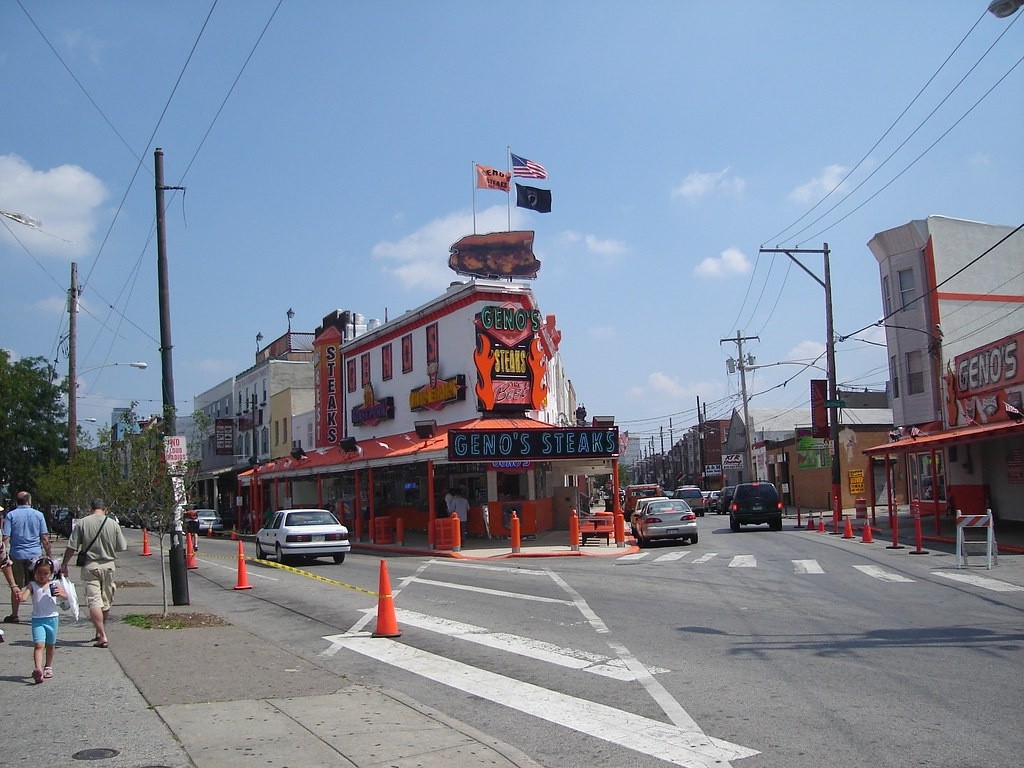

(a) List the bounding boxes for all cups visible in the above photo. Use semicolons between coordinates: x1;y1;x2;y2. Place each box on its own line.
49;582;58;597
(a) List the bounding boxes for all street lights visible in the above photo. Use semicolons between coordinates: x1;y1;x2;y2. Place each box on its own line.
668;427;708;491
68;360;148;466
745;360;842;522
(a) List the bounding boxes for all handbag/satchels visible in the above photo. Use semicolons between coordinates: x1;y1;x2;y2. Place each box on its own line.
76;551;85;567
55;574;79;621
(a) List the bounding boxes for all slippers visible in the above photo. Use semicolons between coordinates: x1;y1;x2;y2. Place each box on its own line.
95;633;100;640
93;642;108;648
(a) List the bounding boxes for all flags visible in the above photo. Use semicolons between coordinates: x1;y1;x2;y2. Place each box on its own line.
1005;403;1018;413
911;426;920;435
476;164;512;193
621;431;629;455
966;415;973;424
261;451;328;472
511;152;547;178
515;183;551;213
356;435;411;458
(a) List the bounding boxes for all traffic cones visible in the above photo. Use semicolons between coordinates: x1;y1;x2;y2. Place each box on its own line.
371;558;403;638
860;514;875;543
185;532;199;569
805;509;819;531
205;520;214;538
815;511;827;532
139;527;153;556
229;522;238;540
233;539;254;590
840;514;856;538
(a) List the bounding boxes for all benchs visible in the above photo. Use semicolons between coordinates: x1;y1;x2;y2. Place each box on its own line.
580;512;613;546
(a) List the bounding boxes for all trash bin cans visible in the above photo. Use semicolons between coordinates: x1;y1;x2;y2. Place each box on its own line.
434;518;452;550
595;512;613;538
375;516;394;544
604;499;613;512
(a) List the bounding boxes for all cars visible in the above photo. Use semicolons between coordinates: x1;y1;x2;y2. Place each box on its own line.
619;487;721;513
182;509;224;536
255;509;351;565
634;498;699;548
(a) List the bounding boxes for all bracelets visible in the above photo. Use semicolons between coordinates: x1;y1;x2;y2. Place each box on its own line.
9;584;18;587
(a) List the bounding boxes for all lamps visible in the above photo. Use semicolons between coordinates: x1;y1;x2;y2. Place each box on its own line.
1006;401;1023;424
247;456;264;468
592;415;614;428
339;436;358;454
414;420;437;447
291;446;308;461
287;307;295;332
890;430;901;443
255;332;263;353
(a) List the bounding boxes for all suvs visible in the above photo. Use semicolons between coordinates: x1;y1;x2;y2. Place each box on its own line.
728;480;784;531
621;483;664;522
668;487;707;516
716;485;736;515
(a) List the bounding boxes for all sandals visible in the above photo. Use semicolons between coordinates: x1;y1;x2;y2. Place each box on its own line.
4;614;19;623
43;665;52;677
32;669;43;684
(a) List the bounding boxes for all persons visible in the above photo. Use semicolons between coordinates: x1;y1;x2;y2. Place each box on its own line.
18;558;68;683
2;492;52;622
435;489;470;547
59;500;127;648
265;508;272;522
0;506;23;601
185;517;200;551
